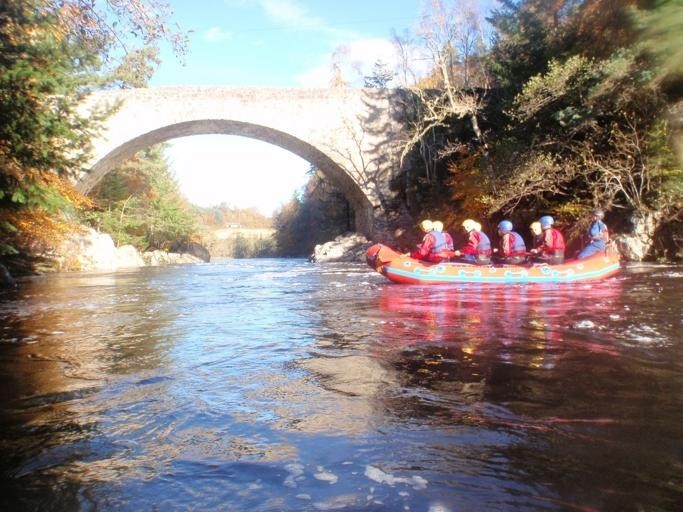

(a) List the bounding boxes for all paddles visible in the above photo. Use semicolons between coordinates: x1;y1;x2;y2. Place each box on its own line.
441;248;465;259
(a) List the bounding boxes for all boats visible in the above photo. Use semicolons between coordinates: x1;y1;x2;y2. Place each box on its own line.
362;241;628;287
380;279;624;316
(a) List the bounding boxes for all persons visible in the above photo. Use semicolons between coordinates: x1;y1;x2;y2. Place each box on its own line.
576;210;608;259
400;215;565;265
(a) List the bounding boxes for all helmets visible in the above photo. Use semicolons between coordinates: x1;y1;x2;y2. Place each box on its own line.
419;210;604;235
